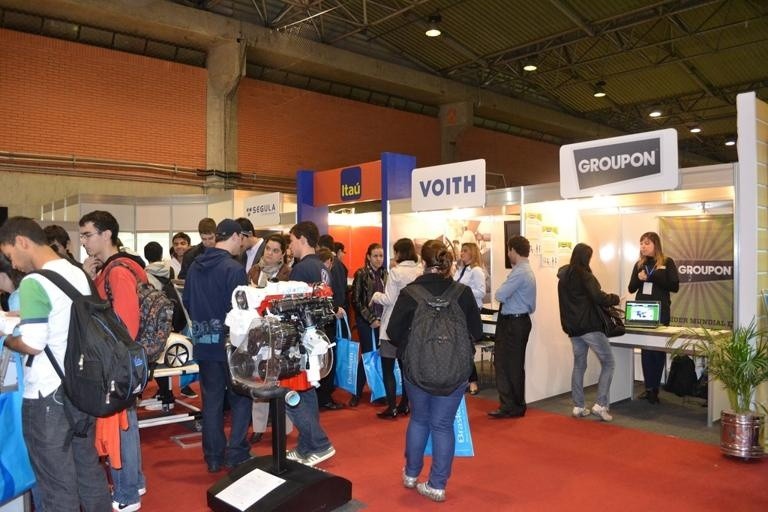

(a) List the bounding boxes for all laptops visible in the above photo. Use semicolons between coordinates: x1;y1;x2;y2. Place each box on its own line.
623;300;662;329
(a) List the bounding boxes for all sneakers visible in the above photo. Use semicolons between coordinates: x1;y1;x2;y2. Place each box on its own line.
138;386;198;411
572;407;590;417
402;464;418;488
591;404;613;421
417;481;446;502
207;432;336;473
109;498;142;512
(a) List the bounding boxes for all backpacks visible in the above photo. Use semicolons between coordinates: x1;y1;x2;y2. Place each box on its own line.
402;281;476;397
25;266;148;415
152;266;187;332
103;261;175;364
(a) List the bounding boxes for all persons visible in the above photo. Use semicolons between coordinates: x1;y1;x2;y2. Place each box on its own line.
368;238;423;419
627;232;678;405
79;210;148;512
0;216;112;512
556;243;625;422
487;233;535;418
0;217;386;410
182;219;256;472
451;242;486;395
247;234;293;445
385;239;482;503
284;221;335;467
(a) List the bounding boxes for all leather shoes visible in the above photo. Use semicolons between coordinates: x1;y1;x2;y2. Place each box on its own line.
321;394;410;418
489;410;506;417
638;391;648;399
469;385;479;395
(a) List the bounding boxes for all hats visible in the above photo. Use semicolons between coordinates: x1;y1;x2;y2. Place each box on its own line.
215;218;252;236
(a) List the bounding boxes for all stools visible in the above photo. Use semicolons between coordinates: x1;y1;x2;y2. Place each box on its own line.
481;345;495;385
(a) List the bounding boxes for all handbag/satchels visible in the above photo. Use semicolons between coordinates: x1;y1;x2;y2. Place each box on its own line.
424;394;475;457
333;337;360;397
361;349;403;403
597;304;625;337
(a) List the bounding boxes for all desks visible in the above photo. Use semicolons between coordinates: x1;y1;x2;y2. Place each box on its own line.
480;313;498;334
135;357;202;435
607;325;733;428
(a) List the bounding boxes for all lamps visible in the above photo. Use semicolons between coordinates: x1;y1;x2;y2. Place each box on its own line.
425;11;442;37
522;55;537;71
593;81;607;97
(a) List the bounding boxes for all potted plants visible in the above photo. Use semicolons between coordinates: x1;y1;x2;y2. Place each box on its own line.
665;313;768;460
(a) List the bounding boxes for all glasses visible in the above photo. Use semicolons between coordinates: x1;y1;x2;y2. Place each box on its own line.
79;230;102;240
